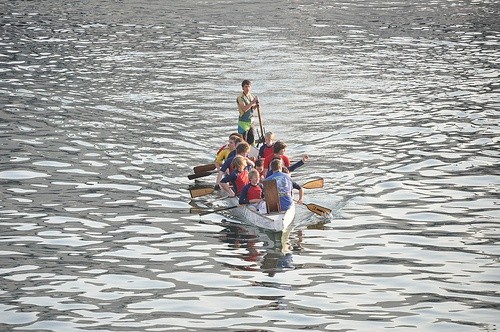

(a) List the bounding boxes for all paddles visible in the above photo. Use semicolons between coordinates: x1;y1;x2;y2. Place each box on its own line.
193;164;226;174
254;96;267;147
187;169;222;180
299;177;324;189
293;199;332;218
190;203;247;216
190;185;224;199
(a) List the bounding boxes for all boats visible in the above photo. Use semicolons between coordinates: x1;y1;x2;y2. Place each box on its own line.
212;137;297;232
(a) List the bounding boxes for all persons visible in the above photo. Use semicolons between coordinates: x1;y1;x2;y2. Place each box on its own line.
236;80;260;148
215;132;309;211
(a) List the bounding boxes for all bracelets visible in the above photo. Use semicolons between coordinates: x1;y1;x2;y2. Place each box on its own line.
252;101;254;104
